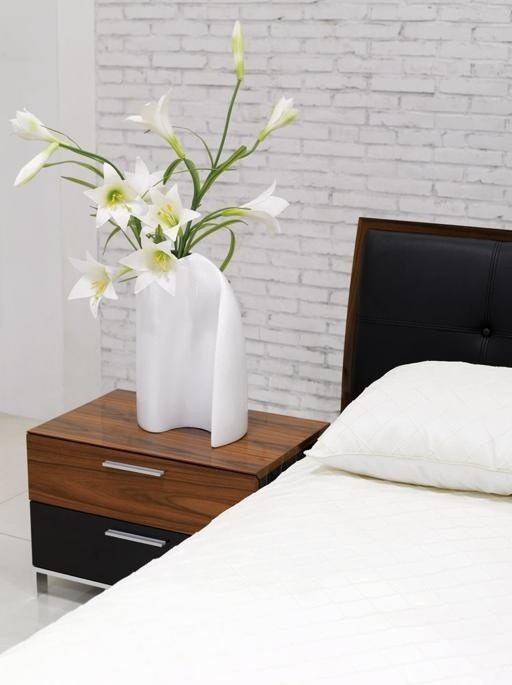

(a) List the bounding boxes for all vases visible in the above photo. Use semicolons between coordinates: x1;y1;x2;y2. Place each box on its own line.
133;248;250;450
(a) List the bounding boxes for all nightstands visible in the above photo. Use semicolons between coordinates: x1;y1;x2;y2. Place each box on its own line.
27;388;331;601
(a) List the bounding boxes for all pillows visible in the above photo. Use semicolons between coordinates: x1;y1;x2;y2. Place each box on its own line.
302;360;511;496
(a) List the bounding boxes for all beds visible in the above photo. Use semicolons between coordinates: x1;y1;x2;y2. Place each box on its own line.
0;216;511;663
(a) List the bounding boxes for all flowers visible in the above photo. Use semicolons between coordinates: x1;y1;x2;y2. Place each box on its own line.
7;18;301;322
